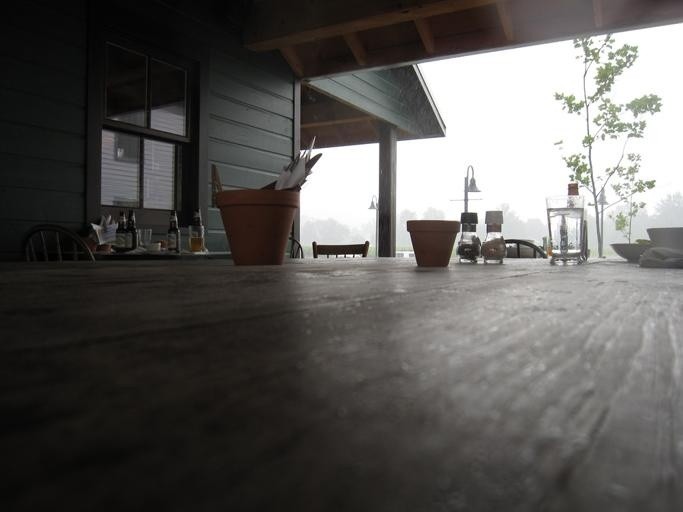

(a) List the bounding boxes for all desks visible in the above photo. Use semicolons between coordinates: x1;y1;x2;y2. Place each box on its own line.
38;248;291;263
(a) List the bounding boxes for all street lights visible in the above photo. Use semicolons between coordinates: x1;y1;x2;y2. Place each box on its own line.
597;187;608;256
463;165;480;213
368;197;379;257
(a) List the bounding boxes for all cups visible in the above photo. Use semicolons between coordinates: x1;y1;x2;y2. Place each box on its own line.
188;225;204;252
84;244;110;254
544;195;586;266
138;229;168;251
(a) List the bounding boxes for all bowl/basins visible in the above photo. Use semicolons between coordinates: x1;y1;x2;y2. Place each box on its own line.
646;226;682;250
610;243;653;263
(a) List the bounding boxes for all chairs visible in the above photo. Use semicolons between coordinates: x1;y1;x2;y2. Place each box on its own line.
502;238;549;258
286;235;304;259
310;239;370;258
16;223;96;264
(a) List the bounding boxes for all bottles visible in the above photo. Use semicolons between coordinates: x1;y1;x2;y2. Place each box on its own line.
567;184;590;262
165;210;181;254
125;210;137;252
480;211;506;264
190;208;203;251
115;212;124;253
456;214;483;264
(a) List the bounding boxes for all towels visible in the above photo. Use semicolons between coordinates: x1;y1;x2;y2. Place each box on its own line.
636;246;682;269
